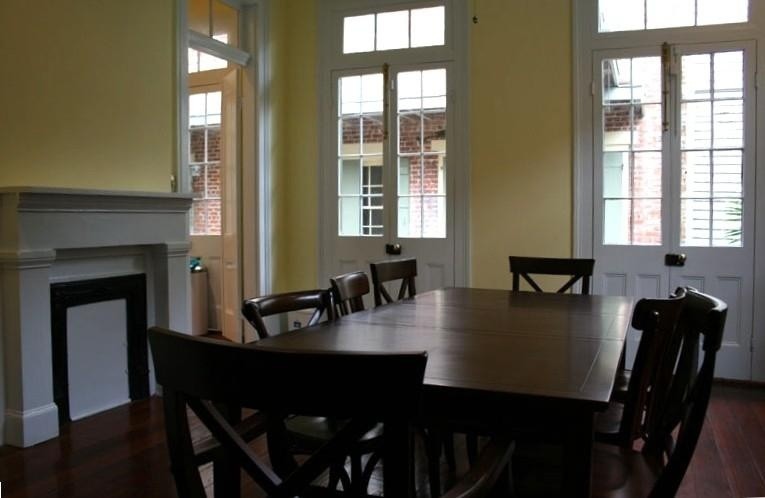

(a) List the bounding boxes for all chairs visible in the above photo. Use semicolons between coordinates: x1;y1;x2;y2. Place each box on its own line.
370;257;417;306
242;290;417;497
578;286;698;439
144;323;430;498
329;271;370;319
509;255;595;295
515;289;730;498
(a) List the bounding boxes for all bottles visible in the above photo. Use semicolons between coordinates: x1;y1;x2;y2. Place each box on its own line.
171;175;176;192
